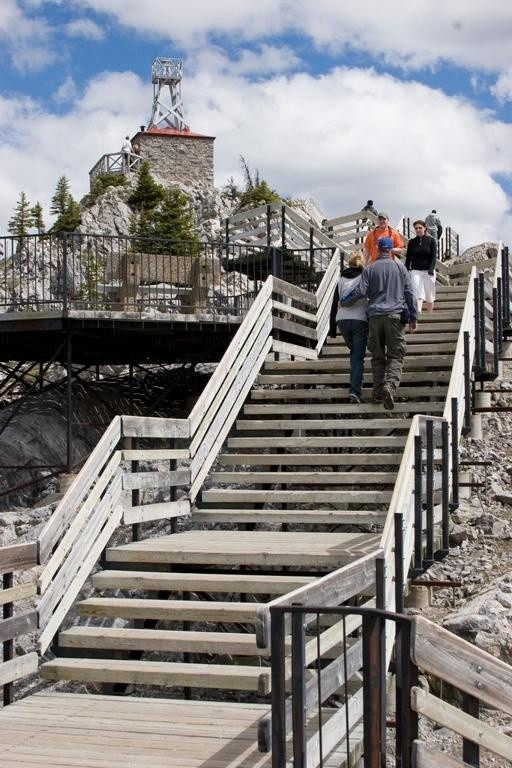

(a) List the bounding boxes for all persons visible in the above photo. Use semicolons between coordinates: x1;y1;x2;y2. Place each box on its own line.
336;236;418;409
361;199;378;231
120;136;134;170
328;251;370;403
363;209;404;267
424;208;443;240
403;219;437;313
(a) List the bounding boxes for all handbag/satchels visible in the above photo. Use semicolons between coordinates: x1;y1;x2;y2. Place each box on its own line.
402;303;411;324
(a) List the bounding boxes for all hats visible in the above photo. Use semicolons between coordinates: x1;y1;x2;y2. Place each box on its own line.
378;238;392;249
377;211;388;220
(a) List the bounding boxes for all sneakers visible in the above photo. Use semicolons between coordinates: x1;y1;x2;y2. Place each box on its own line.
349;390;362;404
371;389;385;404
382;383;394;410
